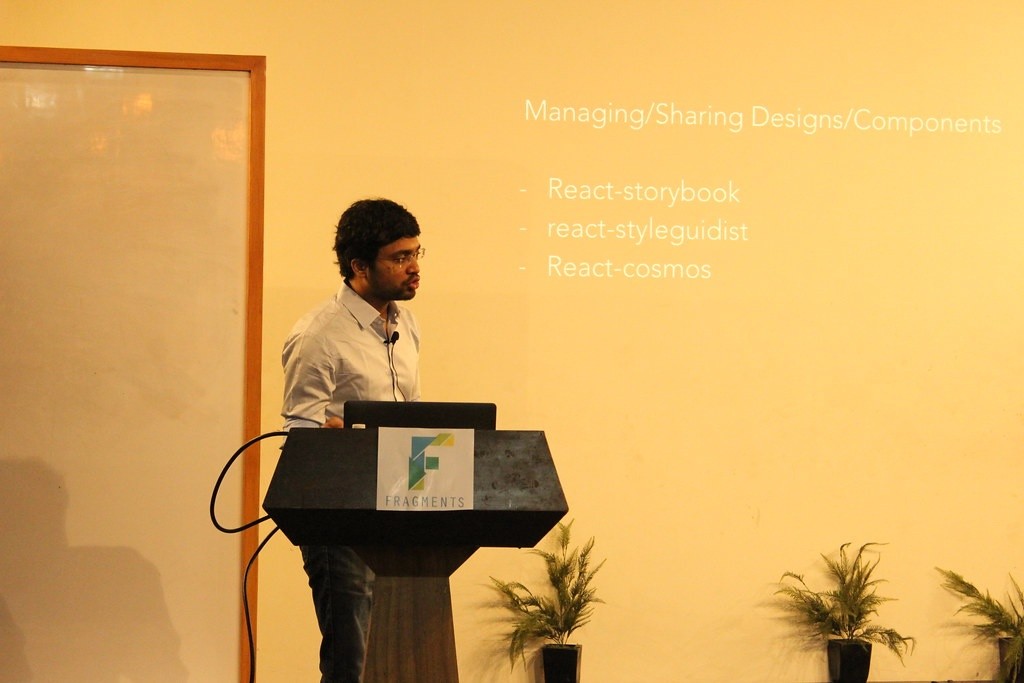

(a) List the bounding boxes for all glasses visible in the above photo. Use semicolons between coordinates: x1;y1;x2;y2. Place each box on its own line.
374;248;426;269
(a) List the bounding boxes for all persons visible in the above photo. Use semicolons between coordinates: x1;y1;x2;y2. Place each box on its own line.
281;197;426;681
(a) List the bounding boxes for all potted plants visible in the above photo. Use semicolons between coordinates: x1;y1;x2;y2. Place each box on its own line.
471;517;605;682
934;565;1024;683
774;540;917;683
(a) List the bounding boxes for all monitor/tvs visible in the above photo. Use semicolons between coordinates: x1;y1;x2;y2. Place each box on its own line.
344;401;497;430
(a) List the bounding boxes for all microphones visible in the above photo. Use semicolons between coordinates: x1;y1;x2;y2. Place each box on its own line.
391;331;399;346
